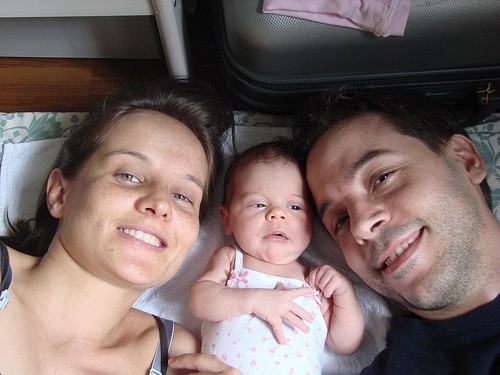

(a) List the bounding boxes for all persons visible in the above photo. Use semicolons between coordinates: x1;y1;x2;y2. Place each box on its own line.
0;91;243;375
291;82;500;375
188;142;364;375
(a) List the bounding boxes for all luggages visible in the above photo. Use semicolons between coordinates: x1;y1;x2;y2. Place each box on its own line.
217;1;500;107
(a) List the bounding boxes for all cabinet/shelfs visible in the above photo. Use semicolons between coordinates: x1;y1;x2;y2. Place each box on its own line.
0;0;190;83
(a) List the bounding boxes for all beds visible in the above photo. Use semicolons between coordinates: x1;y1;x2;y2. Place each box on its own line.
0;110;500;375
